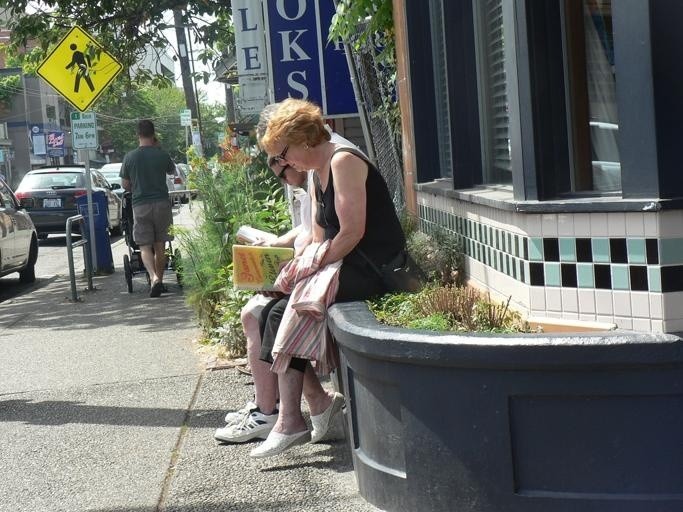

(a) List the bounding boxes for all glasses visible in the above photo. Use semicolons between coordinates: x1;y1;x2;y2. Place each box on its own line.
279;165;289;177
275;145;289;161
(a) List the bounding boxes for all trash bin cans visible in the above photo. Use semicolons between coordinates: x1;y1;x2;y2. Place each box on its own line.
74;190;116;275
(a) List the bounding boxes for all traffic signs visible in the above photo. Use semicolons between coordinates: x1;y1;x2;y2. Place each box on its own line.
70;111;99;150
179;109;192;127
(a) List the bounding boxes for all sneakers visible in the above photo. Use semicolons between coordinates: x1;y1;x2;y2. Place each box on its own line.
215;392;282;443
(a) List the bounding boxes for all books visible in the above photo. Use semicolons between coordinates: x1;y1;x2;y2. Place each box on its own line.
234;247;294;292
234;225;278;246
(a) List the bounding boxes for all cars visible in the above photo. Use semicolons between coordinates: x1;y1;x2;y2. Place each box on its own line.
14;167;123;240
101;160;220;212
0;177;37;282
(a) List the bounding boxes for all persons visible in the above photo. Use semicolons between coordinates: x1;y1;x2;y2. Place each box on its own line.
119;120;176;298
249;99;406;459
235;155;309;376
214;103;370;443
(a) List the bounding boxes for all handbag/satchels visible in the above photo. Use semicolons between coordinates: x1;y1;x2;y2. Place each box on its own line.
363;251;424;291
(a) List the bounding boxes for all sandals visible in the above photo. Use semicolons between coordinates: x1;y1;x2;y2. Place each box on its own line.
249;391;345;458
150;279;163;298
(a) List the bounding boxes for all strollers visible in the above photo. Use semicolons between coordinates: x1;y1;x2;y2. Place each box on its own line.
121;191;184;294
